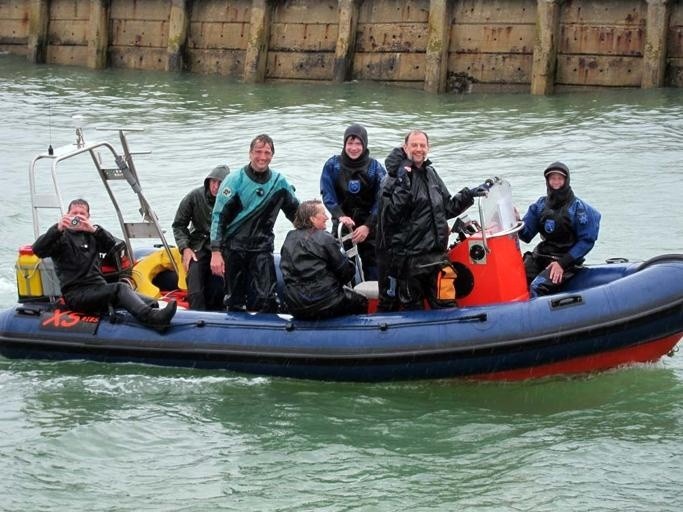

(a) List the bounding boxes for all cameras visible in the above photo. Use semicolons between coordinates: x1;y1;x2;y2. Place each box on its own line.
71;218;81;228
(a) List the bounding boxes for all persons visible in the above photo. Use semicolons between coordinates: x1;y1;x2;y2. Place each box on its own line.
171;164;232;311
279;198;369;324
319;123;386;280
208;132;305;313
30;198;178;336
513;159;602;300
374;129;490;313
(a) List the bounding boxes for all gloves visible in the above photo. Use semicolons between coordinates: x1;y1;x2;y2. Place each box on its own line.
469;183;489;198
398;158;414;187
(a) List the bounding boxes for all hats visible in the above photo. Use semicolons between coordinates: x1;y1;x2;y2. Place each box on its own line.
341;122;369;163
541;159;571;192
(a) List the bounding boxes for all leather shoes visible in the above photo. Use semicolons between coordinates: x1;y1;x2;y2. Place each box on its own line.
141;297;178;336
